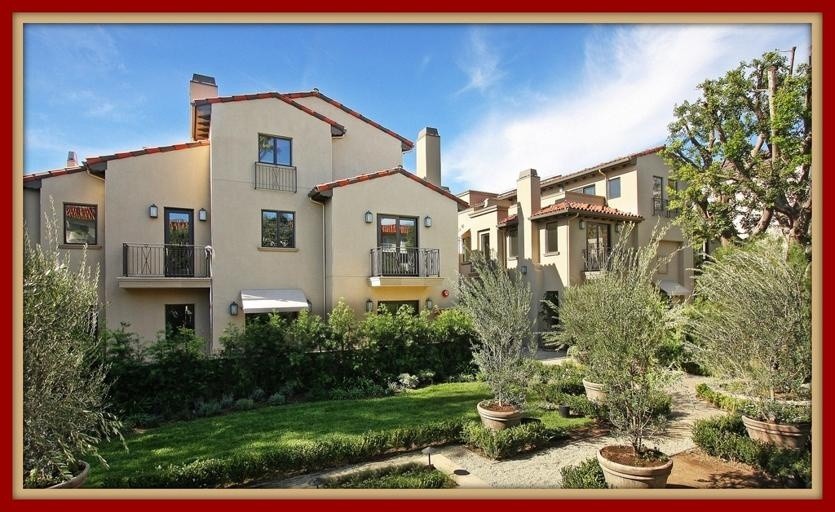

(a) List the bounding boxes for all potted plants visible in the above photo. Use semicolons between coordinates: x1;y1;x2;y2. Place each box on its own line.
581;217;685;400
676;233;811;444
24;191;132;487
549;214;708;487
458;254;538;431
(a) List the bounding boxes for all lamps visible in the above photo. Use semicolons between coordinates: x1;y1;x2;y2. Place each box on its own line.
365;210;373;223
149;203;158;218
366;298;373;312
426;297;432;309
579;220;585;230
199;207;207;222
615;223;622;233
425;216;432;226
230;301;238;316
